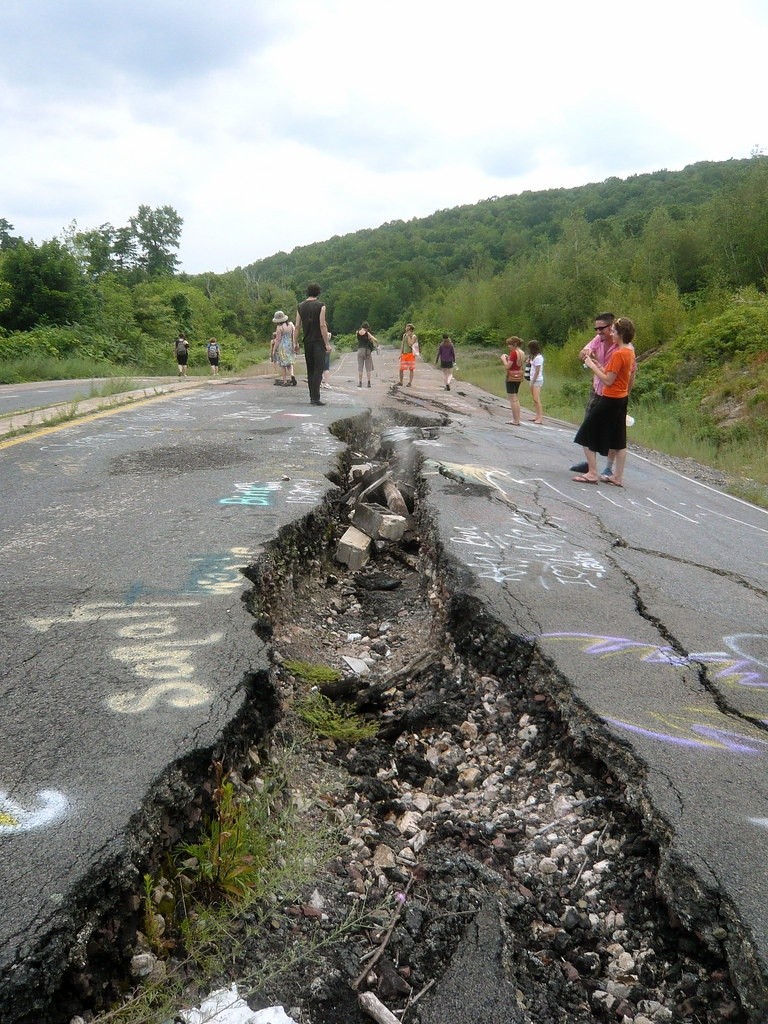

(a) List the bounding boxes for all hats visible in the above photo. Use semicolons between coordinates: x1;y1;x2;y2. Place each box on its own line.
272;311;288;323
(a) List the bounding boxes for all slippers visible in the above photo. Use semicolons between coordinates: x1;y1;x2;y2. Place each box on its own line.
572;475;598;485
600;477;624;487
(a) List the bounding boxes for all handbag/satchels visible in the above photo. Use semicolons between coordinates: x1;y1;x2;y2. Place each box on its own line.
411;335;419;356
366;333;379;356
524;357;530;381
507;370;523;383
453;362;459;371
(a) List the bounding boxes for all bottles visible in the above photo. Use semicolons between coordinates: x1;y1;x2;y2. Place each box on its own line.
626;415;635;426
453;362;458;371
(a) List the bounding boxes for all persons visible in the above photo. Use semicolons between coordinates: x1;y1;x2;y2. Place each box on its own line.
174;334;189;377
396;324;418;386
322;321;331;389
270;311;299;386
207;338;220;376
571;314;637;475
575;316;635;486
528;340;543;424
356;323;377;388
500;336;525;425
294;284;332;406
435;334;456;391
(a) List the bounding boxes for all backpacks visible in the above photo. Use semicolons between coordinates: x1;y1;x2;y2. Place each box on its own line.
208;343;217;358
176;339;186;356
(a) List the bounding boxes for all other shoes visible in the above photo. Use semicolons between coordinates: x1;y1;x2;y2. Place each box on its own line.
446;384;450;391
282;383;288;387
528;418;535;421
357;381;362;387
570;463;589;473
533;421;542;424
292;376;297;386
310;399;325;406
395;382;402;386
601;468;612;477
214;373;219;375
505;421;520;426
406;383;411;387
367;381;371;387
178;372;182;377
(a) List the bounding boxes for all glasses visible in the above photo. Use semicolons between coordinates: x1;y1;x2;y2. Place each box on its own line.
594;323;612;331
